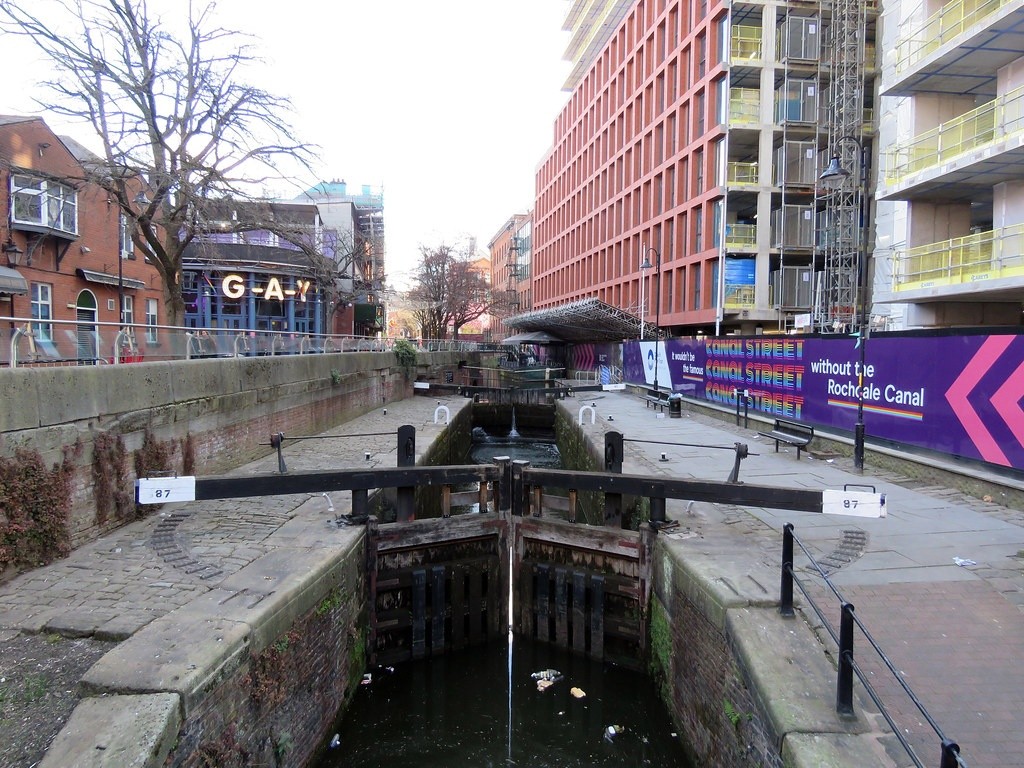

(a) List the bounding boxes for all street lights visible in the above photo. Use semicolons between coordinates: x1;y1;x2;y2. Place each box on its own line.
116;175;153;331
639;247;661;389
818;134;868;471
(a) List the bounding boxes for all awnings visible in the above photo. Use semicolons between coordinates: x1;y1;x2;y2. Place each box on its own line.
0;266;28;296
76;267;146;289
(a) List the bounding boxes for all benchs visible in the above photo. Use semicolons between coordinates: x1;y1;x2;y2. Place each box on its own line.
757;418;814;460
639;389;670;413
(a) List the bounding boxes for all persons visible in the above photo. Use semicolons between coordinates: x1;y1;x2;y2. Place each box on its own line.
185;329;218;360
517;353;527;367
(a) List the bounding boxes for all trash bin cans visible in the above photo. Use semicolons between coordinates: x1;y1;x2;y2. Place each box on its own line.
668;397;681;418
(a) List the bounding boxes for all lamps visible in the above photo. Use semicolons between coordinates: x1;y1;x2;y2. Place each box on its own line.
39;142;52;148
81;245;90;253
